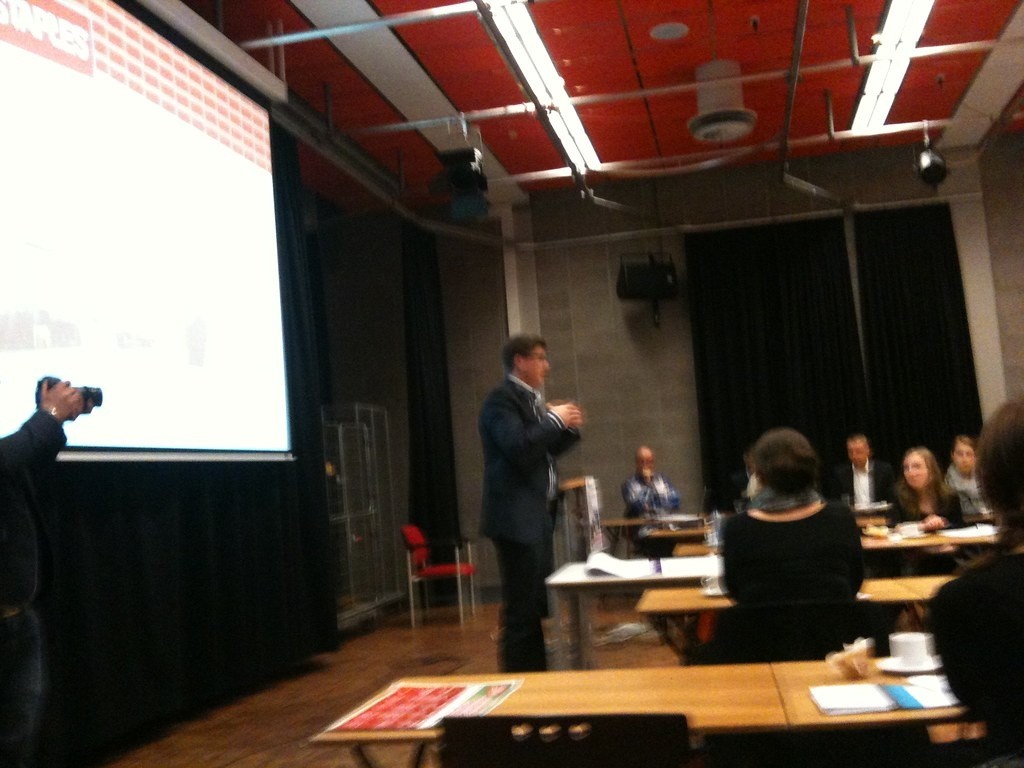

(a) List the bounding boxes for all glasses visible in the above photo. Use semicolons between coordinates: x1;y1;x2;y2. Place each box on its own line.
522;349;548;365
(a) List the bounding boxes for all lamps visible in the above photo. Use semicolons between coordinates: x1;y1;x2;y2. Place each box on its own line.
852;1;939;134
471;0;603;182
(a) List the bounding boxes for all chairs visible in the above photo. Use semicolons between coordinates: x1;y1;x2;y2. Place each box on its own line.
401;524;475;626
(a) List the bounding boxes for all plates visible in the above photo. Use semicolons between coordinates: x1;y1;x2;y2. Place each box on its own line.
876;657;943;675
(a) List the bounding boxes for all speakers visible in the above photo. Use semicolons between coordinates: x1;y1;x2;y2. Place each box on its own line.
615;263;681;303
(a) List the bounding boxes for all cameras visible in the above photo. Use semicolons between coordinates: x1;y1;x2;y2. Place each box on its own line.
35;377;102;420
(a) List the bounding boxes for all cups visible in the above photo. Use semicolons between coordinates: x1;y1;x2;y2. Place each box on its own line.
889;632;929;669
901;524;918;536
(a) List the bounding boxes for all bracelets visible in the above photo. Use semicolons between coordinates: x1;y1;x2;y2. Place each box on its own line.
43;405;58;419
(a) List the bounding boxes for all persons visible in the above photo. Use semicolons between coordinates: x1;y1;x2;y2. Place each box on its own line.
0;378;102;768
480;336;586;674
875;447;964;656
622;446;688;562
930;393;1024;768
825;433;893;508
722;430;867;664
945;431;992;522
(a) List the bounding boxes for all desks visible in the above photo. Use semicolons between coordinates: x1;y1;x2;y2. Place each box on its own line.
311;500;1006;767
